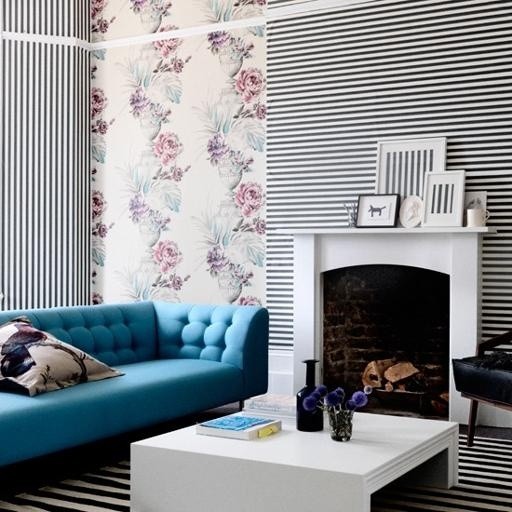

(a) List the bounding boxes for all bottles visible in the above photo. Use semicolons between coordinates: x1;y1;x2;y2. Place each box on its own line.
296;360;324;432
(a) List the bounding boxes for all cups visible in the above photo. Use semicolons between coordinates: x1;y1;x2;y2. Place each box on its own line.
348;212;357;227
467;208;489;227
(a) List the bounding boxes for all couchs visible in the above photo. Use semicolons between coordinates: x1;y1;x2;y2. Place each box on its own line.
0;298;269;512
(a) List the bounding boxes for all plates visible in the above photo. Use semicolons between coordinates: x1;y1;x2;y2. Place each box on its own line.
399;196;424;228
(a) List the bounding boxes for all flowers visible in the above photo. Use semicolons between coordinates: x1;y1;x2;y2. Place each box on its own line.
303;383;369;436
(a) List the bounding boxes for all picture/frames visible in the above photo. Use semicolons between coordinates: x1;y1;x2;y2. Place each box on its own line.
354;137;488;228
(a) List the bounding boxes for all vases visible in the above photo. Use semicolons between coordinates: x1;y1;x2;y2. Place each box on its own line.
295;358;354;444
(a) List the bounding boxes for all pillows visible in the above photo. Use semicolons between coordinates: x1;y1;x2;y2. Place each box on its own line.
3;314;127;400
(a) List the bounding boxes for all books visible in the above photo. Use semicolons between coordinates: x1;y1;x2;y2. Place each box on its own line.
195;418;282;441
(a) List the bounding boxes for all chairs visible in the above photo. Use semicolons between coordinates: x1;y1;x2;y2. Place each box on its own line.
451;330;512;447
